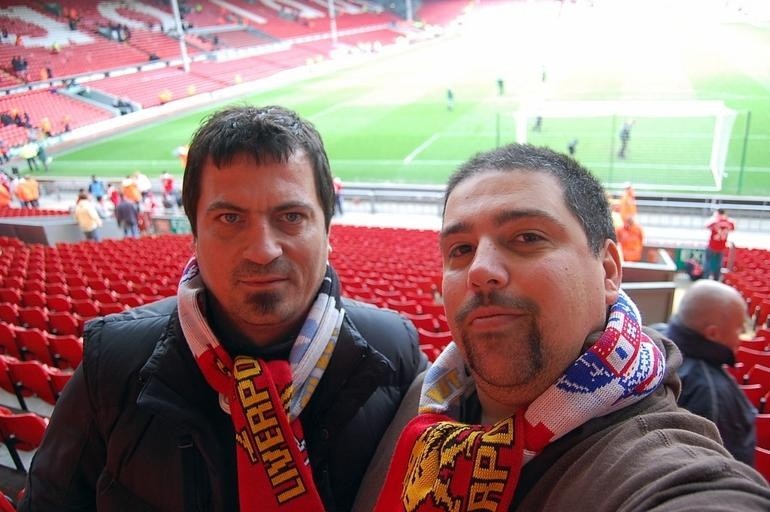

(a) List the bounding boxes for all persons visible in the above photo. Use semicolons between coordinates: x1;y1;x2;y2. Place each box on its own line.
0;0;738;284
350;144;770;512
17;104;431;512
646;278;759;467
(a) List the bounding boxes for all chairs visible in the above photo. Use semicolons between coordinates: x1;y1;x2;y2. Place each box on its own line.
0;201;770;511
0;0;511;159
